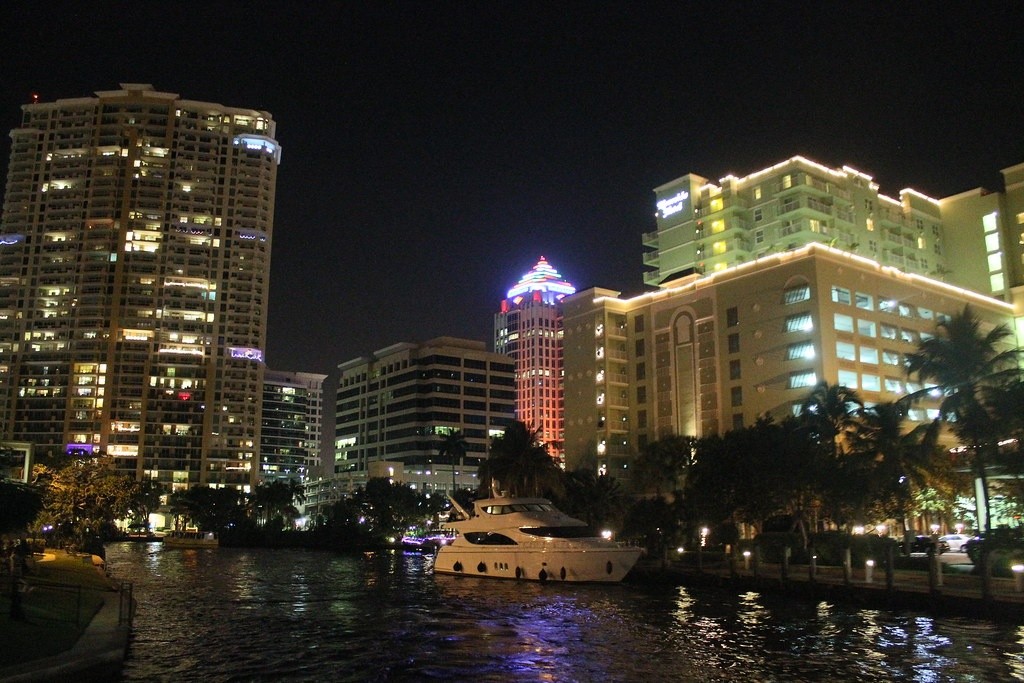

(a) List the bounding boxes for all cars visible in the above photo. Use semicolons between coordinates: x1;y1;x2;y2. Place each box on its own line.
899;532;975;553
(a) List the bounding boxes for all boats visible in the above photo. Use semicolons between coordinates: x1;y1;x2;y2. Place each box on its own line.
162;529;219;549
433;474;641;584
